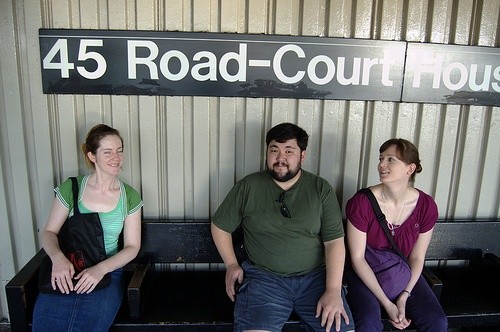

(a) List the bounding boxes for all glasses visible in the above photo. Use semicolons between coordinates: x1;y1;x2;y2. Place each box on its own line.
275;191;291;219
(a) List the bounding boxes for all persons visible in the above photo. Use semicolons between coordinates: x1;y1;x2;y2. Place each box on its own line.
31;124;144;332
207;122;355;332
344;141;449;332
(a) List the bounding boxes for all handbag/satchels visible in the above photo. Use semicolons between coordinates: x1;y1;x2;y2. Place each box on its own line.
39;177;112;292
365;243;411;298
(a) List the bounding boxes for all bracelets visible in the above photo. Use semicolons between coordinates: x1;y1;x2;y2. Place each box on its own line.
403;289;411;297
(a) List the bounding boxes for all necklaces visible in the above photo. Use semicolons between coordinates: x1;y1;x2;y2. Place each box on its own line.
382;192;406;236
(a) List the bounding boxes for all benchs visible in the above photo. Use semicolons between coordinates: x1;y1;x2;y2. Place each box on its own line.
5;221;500;332
